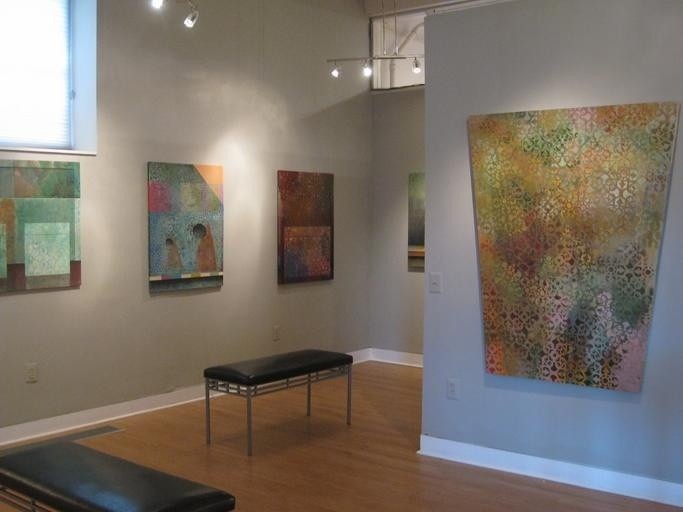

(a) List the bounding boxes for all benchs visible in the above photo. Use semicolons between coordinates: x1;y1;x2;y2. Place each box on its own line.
204;350;354;456
0;441;235;512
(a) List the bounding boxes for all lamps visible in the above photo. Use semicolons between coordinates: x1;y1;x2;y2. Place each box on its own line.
184;11;199;28
331;58;422;78
